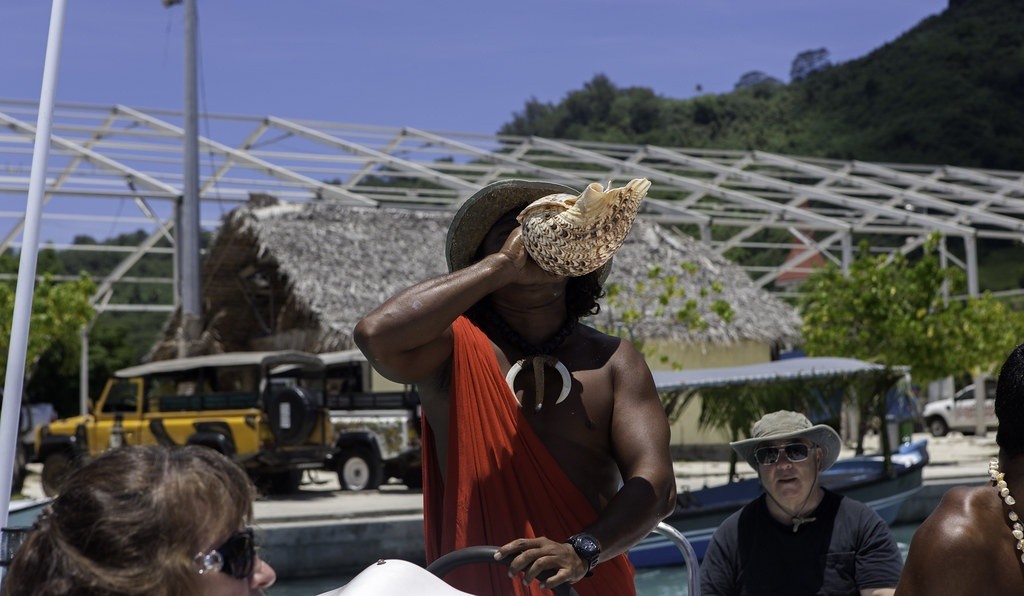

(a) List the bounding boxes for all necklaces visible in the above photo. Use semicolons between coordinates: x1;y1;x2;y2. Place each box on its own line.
987;458;1024;563
488;304;573;409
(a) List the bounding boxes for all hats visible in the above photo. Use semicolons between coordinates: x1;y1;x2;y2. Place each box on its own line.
728;409;842;473
445;180;612;288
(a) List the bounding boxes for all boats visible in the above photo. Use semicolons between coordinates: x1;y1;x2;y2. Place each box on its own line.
609;358;933;569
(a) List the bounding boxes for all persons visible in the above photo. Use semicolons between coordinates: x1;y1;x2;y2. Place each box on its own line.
699;409;904;596
0;439;276;596
896;344;1024;596
353;178;677;596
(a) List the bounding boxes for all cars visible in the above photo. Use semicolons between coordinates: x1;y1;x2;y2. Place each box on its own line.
923;374;1000;437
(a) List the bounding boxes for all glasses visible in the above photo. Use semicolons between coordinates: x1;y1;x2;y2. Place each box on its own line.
188;528;255;580
753;443;816;466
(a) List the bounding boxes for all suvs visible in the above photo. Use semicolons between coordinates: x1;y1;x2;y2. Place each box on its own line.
260;347;427;493
34;350;334;499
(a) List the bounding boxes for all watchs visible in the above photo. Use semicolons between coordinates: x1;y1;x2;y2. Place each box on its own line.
567;533;600;572
